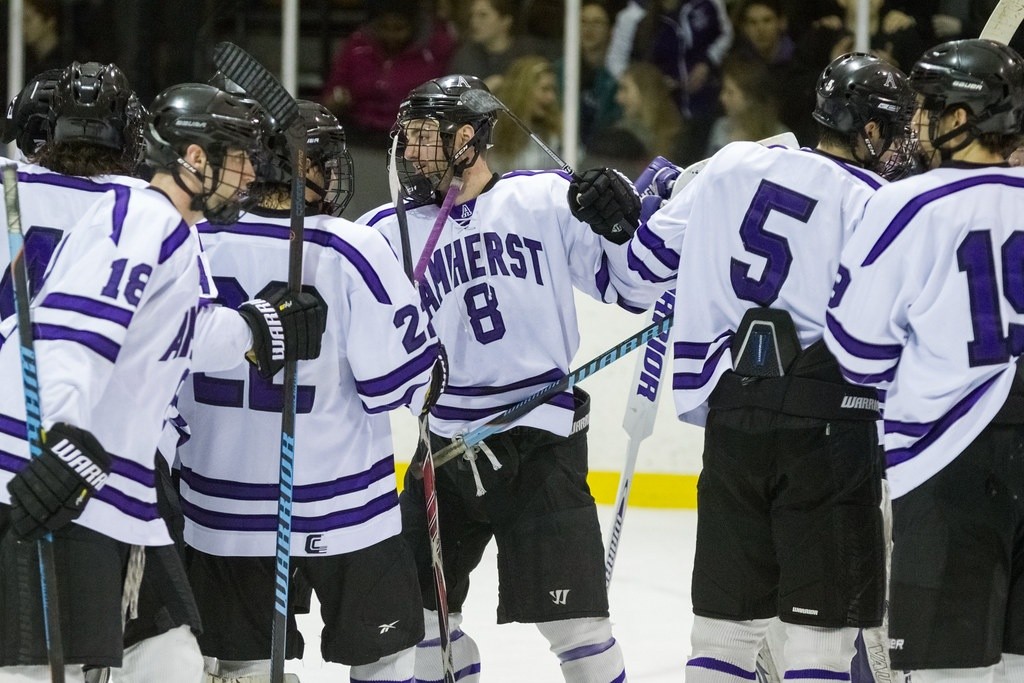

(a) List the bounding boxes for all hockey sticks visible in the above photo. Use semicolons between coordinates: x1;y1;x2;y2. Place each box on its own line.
404;311;674;557
0;161;70;683
978;0;1024;45
599;128;803;590
208;37;312;683
381;130;462;683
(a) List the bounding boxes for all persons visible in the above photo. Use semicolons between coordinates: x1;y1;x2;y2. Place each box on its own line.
822;41;1024;683
0;0;1024;683
347;72;679;683
628;49;920;681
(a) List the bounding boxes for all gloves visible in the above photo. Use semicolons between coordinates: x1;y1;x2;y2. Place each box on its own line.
237;279;328;378
5;421;114;541
418;344;450;422
568;166;643;246
631;155;679;199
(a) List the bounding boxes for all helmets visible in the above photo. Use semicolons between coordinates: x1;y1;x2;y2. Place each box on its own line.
386;74;500;204
50;59;143;174
248;98;355;217
3;68;63;163
906;38;1024;171
145;82;278;224
207;70;277;136
811;51;917;176
